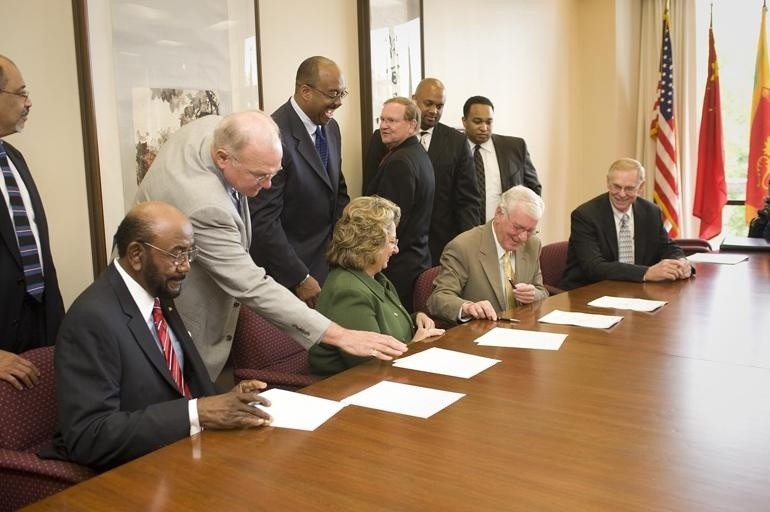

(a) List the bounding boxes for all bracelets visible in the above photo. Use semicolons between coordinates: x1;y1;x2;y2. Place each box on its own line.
298;275;309;286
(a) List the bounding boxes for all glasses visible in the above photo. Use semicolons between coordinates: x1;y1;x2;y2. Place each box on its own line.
222;148;283;185
139;240;198;266
306;83;348;102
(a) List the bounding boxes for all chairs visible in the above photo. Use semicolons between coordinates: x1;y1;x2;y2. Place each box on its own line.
228;290;324;393
536;240;573;293
675;238;713;255
414;260;447;328
0;341;91;511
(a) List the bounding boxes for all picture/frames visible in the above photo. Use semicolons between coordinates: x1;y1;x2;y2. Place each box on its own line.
71;0;267;284
355;0;429;201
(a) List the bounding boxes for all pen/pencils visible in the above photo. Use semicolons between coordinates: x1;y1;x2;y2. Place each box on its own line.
508;279;516;289
497;316;521;322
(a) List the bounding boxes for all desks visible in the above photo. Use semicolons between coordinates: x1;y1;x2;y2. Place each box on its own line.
13;246;768;512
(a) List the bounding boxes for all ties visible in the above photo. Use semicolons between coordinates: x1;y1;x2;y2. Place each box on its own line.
504;252;517;309
151;296;192;401
0;139;45;304
619;215;633;264
314;126;328;168
473;145;486;224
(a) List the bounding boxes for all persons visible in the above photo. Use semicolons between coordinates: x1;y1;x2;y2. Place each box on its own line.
362;78;482;268
308;194;445;377
0;56;67;393
427;185;550;326
558;159;696;290
364;97;436;314
108;109;408;394
248;56;351;308
36;201;272;472
461;96;542;224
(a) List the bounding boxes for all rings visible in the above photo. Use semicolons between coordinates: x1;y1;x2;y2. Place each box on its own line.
372;350;376;355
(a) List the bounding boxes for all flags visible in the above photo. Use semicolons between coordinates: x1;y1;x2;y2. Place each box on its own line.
746;7;770;225
692;26;728;240
648;11;681;241
